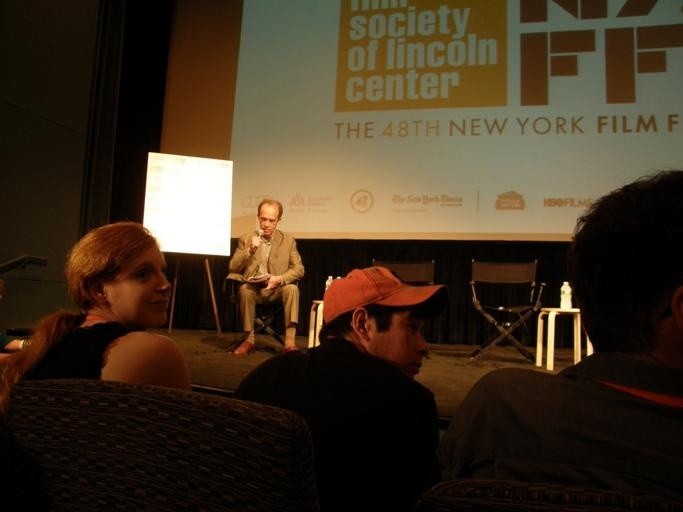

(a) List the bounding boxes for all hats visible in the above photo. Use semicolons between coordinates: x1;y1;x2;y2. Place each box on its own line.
322;265;447;326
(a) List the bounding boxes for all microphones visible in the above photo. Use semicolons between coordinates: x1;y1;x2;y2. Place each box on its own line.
250;229;265;254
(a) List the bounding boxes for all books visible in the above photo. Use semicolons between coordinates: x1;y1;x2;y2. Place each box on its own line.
242;271;271;283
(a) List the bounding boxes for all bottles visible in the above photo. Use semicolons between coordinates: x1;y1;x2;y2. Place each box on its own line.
560;282;573;308
325;276;341;291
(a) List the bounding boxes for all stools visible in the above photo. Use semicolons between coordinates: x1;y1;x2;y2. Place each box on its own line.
536;307;594;371
307;300;324;348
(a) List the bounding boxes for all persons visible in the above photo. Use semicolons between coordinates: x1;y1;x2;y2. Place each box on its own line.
439;170;682;508
224;198;308;356
1;217;189;403
228;267;447;512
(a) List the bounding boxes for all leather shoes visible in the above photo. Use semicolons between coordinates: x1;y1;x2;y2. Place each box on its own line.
281;343;299;353
233;338;256;358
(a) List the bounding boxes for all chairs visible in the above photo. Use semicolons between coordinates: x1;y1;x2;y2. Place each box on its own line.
372;259;435;287
226;280;301;352
470;257;546;362
1;379;325;510
408;477;683;511
